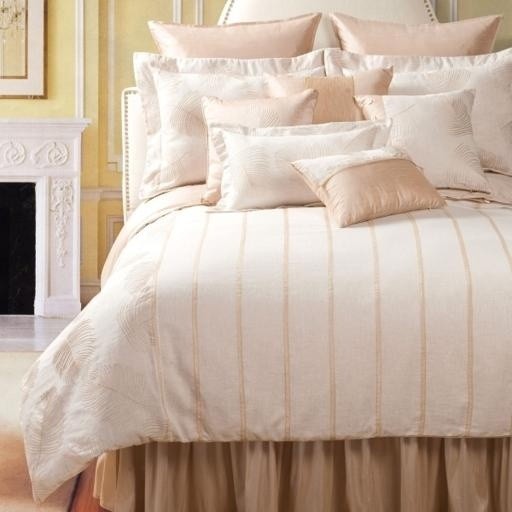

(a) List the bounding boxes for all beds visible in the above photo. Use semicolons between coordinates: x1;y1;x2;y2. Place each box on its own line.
88;2;511;511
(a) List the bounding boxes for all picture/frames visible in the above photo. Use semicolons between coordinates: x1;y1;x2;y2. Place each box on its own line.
1;1;49;100
